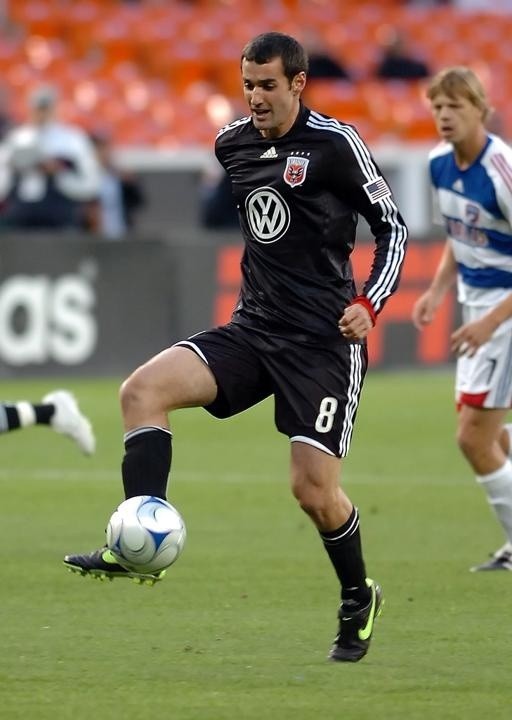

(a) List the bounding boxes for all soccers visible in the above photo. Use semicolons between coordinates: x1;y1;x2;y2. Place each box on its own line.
106;495;185;574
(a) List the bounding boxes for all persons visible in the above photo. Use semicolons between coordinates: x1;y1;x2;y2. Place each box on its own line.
0;81;142;236
0;388;93;456
63;30;410;662
200;168;238;227
380;32;425;78
411;65;512;571
301;31;345;78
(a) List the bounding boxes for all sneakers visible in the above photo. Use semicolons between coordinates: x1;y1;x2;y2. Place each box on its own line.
330;577;384;661
43;389;96;455
468;546;512;571
63;545;167;587
